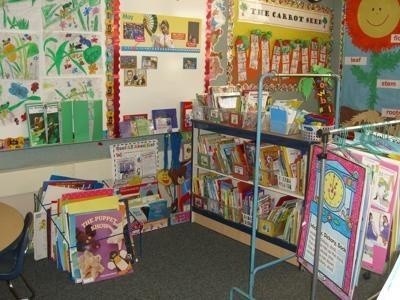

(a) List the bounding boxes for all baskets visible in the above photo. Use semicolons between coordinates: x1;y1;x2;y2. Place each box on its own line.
301;124;336;142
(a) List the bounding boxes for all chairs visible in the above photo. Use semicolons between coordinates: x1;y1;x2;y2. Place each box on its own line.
0;211;34;299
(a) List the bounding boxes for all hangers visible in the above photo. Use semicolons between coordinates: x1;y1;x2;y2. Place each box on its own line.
330;119;400;175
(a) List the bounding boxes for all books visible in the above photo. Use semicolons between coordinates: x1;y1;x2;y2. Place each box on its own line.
29;174;135;288
197;133;308;196
193;171;304;246
197;86;307;135
109;137;191;232
25;99;195;148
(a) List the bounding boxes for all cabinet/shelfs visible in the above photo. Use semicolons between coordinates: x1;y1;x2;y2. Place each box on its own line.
190;118;320;267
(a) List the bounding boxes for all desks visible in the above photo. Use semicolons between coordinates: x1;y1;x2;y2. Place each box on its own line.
1;202;25;253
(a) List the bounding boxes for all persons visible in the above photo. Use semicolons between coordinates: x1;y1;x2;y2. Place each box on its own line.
125;70;137;86
137;72;145;85
143;17;175;49
183;58;194;69
